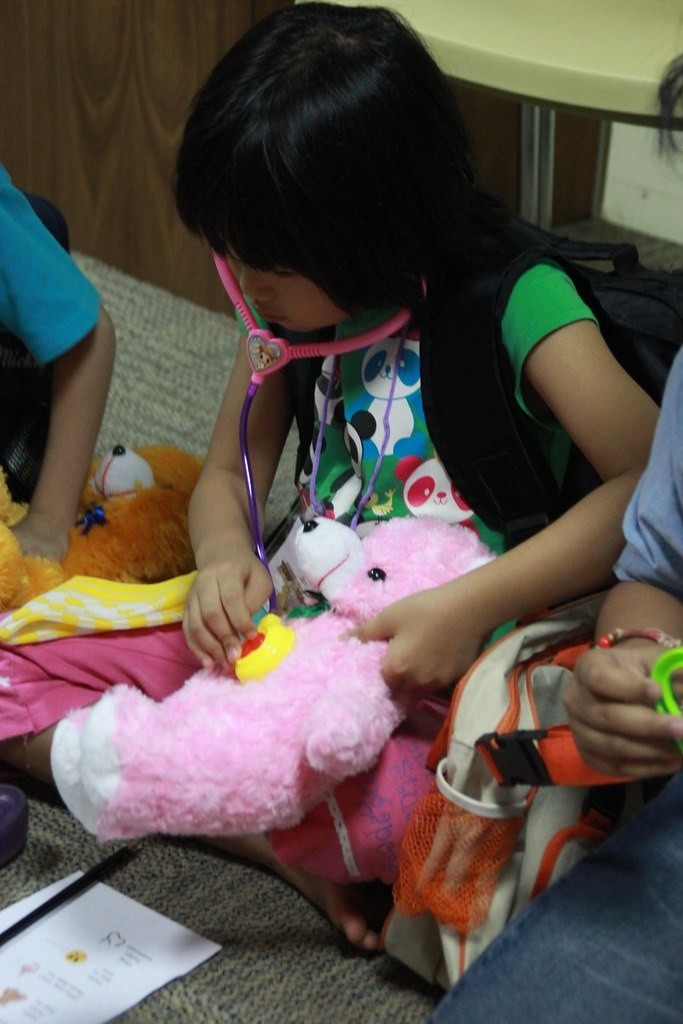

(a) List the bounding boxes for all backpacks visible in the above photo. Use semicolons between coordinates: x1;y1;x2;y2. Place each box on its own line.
266;194;682;611
0;192;71;504
379;589;674;993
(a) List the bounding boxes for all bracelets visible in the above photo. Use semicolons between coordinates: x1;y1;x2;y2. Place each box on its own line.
594;626;681;648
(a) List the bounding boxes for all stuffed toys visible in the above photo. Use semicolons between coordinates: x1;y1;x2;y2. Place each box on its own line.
50;515;500;843
0;444;205;616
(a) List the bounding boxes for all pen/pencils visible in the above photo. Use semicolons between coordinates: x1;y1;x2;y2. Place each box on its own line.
0;833;163;946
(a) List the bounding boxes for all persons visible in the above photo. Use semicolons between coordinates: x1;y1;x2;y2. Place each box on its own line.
0;0;661;954
0;161;117;566
429;55;683;1024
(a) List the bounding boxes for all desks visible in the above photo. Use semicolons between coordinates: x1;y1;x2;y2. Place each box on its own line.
293;1;683;232
